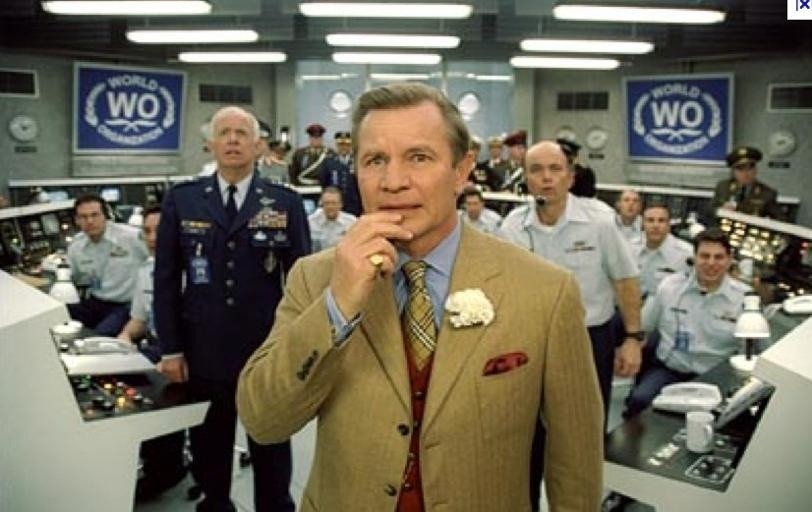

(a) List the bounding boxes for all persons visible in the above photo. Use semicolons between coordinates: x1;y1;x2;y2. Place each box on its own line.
234;82;605;512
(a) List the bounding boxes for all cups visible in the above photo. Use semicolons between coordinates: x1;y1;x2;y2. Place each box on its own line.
686;411;713;454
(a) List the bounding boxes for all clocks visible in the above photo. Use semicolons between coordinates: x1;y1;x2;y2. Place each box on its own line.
555;125;575;142
584;125;607;150
769;129;796;157
10;114;38;141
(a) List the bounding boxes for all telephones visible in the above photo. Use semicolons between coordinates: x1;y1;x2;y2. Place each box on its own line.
783;293;812;315
651;381;723;413
72;336;132;353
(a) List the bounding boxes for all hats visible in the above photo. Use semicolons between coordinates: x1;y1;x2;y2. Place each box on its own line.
334;131;352;144
268;138;292;154
306;123;325;138
553;128;585;159
255;119;272;138
724;145;765;168
469;128;528;151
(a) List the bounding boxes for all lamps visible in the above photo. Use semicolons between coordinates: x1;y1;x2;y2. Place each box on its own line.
41;0;725;81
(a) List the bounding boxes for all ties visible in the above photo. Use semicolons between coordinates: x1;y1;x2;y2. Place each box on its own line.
223;183;240;225
399;258;439;375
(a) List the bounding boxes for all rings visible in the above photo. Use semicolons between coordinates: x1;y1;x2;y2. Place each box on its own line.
369;255;383;271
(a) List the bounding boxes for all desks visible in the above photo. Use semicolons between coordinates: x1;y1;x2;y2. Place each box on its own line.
594;180;812;512
0;192;211;512
289;184;324;215
473;189;534;221
